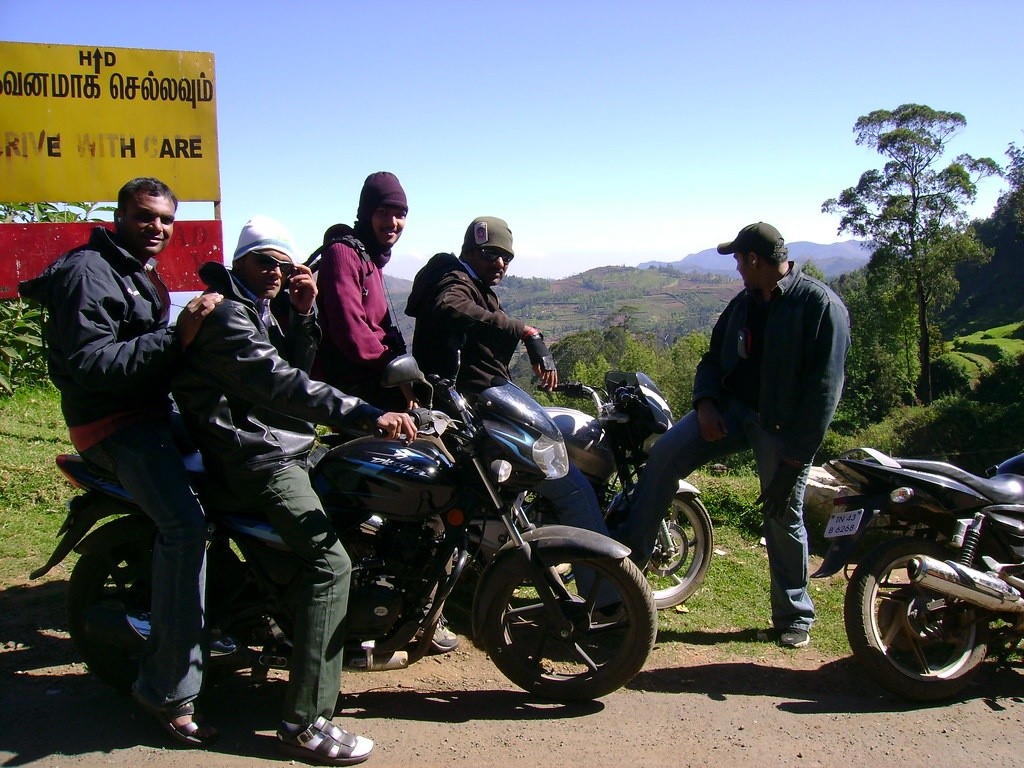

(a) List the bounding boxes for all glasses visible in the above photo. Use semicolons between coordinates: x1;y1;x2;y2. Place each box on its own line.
478;246;513;262
243;253;292;274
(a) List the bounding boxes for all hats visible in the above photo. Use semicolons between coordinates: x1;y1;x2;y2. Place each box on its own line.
357;172;408;223
717;222;787;255
232;218;298;270
461;216;515;257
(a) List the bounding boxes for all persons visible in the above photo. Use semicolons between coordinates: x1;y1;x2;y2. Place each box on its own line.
171;171;626;766
609;222;852;648
18;179;224;746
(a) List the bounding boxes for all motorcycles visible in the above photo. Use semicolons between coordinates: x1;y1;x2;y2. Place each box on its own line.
456;369;715;612
808;446;1024;702
29;356;661;701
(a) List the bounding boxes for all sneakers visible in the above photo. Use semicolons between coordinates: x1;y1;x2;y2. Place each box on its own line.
779;628;810;647
594;602;629;622
415;610;458;653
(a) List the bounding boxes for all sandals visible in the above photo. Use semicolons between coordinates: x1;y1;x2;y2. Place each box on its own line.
275;715;374;766
142;702;217;744
